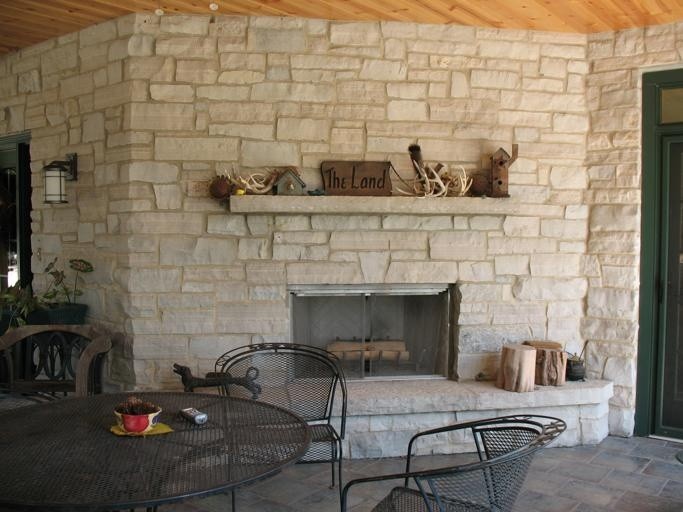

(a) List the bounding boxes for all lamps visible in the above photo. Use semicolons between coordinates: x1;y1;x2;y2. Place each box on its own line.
42;152;77;205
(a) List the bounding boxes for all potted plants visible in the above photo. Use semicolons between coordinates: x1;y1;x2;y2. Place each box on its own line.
1;258;94;325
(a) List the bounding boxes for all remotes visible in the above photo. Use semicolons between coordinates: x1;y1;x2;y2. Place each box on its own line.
180;406;207;425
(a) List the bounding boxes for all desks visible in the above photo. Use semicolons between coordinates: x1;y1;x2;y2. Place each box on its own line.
0;392;316;512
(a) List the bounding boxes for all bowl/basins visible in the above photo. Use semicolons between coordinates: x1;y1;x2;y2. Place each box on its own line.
112;404;163;433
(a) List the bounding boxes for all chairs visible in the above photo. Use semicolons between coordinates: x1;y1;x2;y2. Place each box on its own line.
2;323;112;403
341;414;566;511
214;342;348;511
